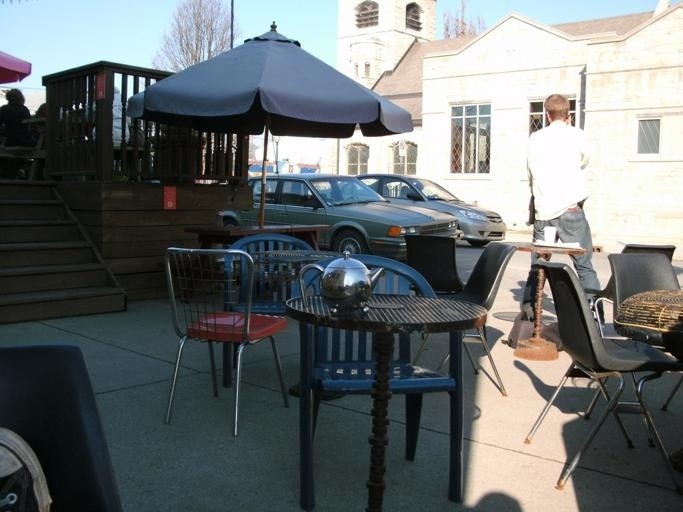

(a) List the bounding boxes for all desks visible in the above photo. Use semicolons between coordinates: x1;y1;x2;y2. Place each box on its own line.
20;117;87;182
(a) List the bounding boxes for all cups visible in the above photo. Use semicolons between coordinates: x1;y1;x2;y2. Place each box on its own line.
543;225;556;243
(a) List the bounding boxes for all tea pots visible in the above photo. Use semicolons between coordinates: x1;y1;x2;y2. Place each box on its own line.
297;249;385;315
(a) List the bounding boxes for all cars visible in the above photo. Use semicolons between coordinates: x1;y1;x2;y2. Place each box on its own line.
219;170;458;261
347;170;506;249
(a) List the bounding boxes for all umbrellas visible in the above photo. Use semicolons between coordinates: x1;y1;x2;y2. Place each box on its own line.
0;50;32;85
123;21;413;227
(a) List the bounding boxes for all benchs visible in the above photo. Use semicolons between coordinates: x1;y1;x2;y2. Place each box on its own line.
0;146;157;183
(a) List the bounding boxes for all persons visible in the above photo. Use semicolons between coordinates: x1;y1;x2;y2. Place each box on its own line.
524;94;604;323
31;100;47;146
129;117;147;160
89;82;131;174
0;89;34;177
